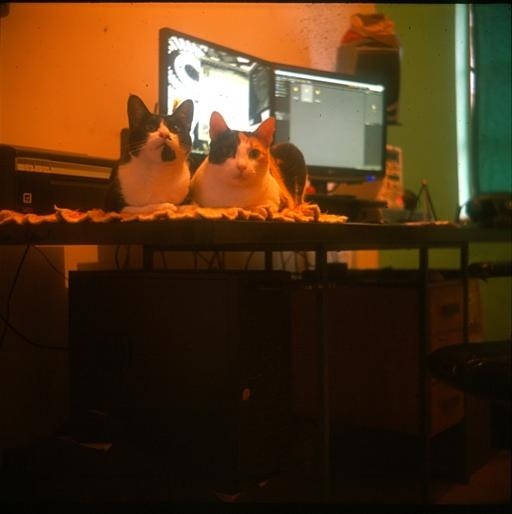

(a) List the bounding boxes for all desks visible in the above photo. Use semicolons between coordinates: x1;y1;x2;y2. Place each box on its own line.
0;220;512;514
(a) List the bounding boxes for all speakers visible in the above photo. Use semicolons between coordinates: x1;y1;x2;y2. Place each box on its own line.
334;45;402;127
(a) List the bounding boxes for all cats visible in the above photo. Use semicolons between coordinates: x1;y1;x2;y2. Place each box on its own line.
189;111;307;217
103;92;197;214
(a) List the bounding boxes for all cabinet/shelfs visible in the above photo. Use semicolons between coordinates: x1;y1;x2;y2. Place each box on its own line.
291;266;482;429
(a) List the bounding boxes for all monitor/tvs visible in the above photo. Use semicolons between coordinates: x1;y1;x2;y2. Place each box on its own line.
273;60;388;195
156;25;278;164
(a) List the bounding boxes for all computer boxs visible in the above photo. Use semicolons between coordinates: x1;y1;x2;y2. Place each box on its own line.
327;143;407;213
